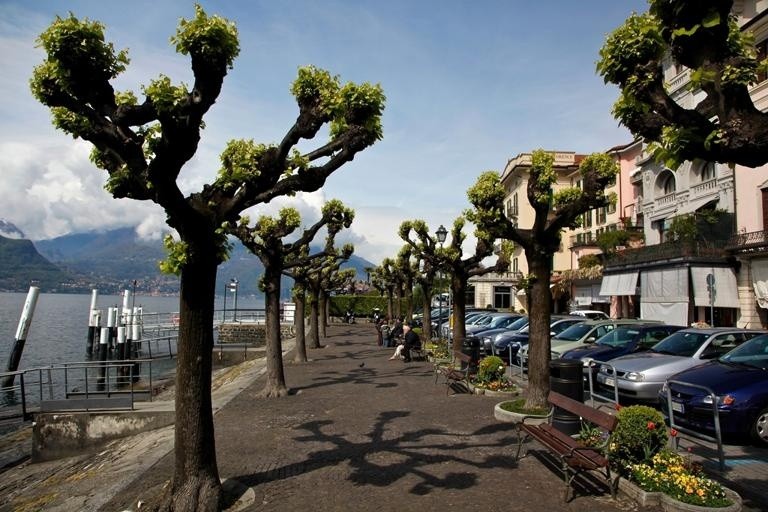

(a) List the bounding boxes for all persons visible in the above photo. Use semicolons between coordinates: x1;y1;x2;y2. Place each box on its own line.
373;312;421;363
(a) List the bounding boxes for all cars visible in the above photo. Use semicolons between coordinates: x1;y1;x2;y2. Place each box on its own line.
561;324;768;449
468;309;664;368
433;293;453;308
401;306;527;341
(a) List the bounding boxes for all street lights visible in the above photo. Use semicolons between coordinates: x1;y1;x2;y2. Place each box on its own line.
435;224;448;344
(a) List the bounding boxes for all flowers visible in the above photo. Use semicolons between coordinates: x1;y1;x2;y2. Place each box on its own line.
627;418;724;502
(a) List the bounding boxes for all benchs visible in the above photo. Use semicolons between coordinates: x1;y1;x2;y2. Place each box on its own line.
408;336;470;397
514;389;619;504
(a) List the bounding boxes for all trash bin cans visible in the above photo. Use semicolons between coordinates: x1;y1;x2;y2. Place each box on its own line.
460;337;480;374
548;358;583;437
